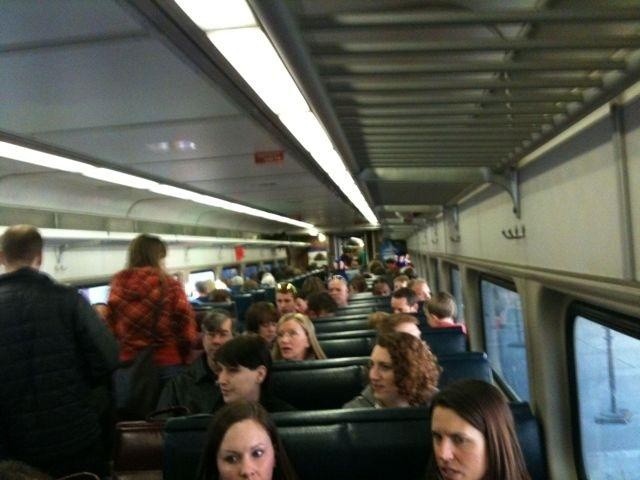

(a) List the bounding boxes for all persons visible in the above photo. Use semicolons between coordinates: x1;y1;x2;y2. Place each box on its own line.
91;303;107;319
423;378;531;480
381;314;422;341
158;308;288;420
342;333;444;409
270;313;327;361
210;334;294;415
193;399;300;480
0;223;121;479
193;236;468;327
106;234;198;418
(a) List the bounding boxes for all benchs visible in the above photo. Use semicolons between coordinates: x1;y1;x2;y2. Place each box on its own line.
165;265;551;476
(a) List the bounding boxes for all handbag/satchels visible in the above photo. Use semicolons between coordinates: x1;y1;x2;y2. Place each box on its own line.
111;347;160;410
